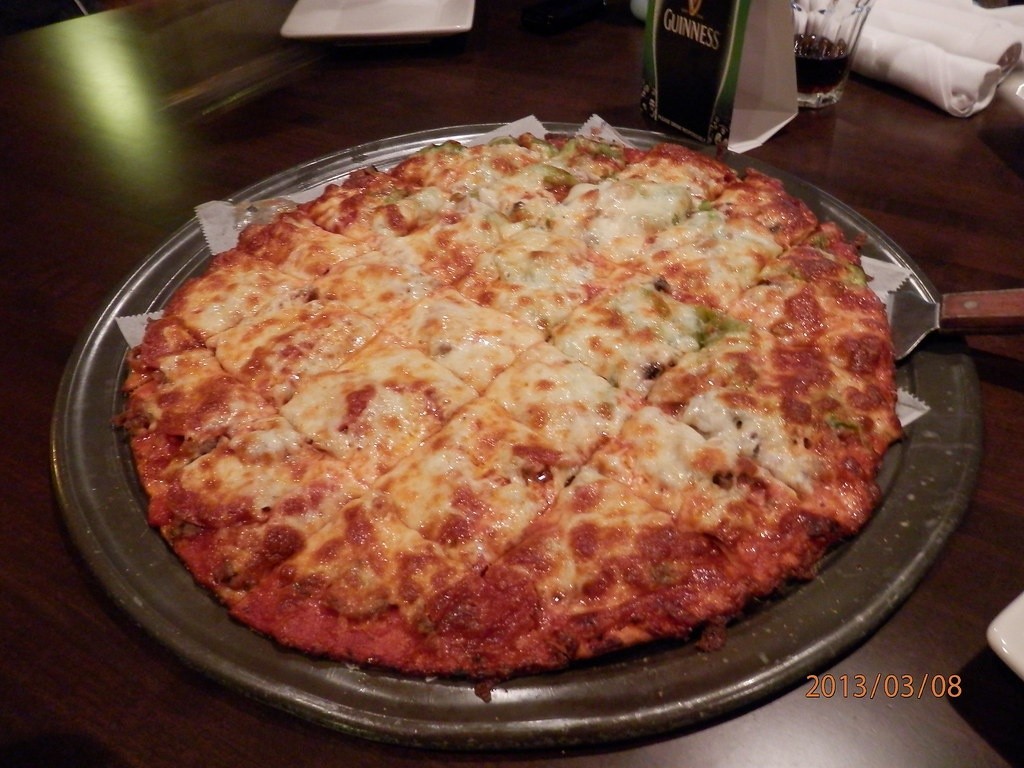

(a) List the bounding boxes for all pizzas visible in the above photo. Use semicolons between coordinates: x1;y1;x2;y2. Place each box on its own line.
118;132;908;680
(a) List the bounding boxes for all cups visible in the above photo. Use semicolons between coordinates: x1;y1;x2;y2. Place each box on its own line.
791;0;876;110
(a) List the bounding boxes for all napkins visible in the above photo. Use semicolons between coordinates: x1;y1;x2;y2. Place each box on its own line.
630;0;1024;117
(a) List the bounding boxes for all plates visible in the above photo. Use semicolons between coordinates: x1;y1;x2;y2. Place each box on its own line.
280;0;476;46
49;115;983;738
